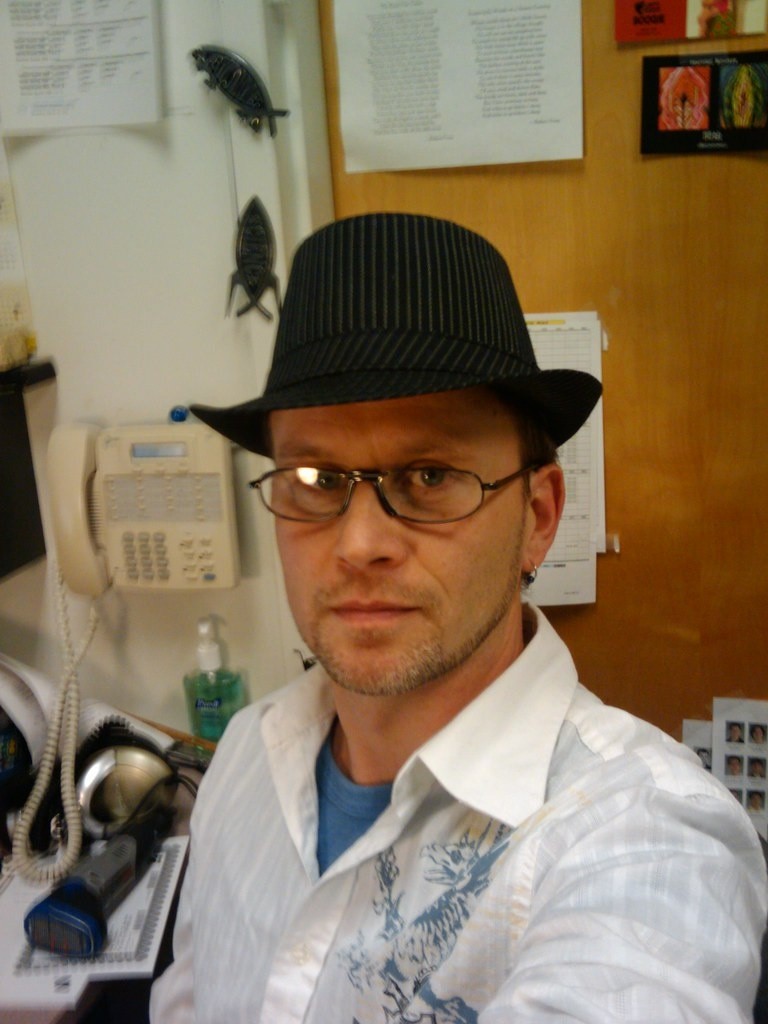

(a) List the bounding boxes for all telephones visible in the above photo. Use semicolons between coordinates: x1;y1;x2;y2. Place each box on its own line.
39;416;248;597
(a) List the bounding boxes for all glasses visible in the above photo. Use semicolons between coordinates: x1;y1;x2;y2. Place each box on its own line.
249;467;529;524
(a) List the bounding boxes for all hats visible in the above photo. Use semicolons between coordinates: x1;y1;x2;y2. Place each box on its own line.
189;213;603;459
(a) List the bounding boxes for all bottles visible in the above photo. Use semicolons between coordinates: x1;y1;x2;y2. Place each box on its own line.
183;621;242;767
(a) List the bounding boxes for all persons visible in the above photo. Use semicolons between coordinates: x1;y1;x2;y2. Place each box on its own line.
149;213;768;1024
694;720;766;815
